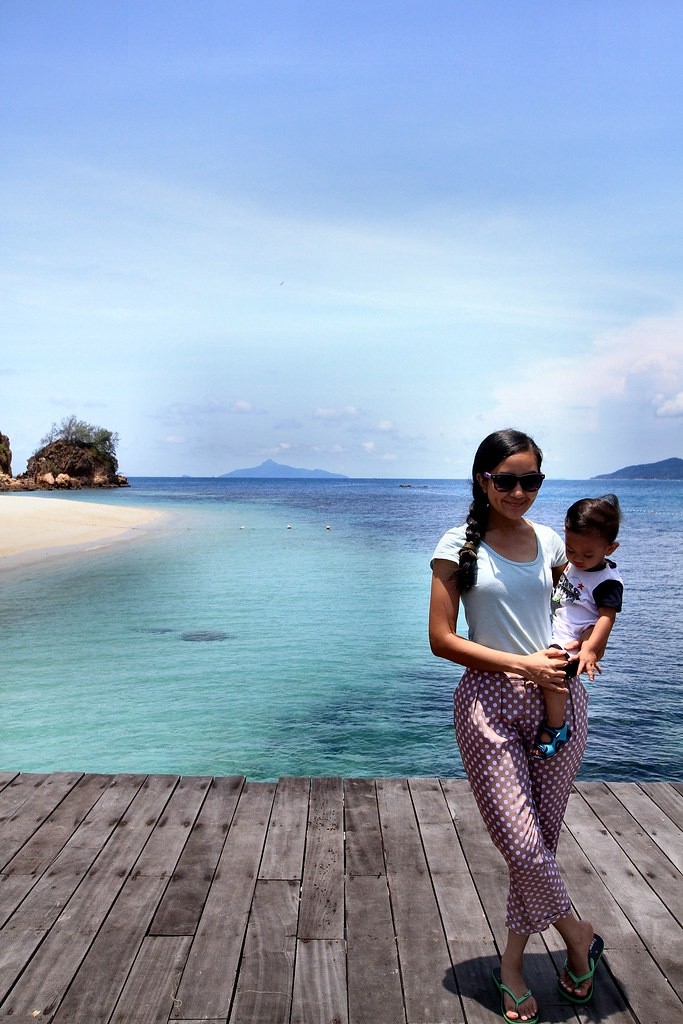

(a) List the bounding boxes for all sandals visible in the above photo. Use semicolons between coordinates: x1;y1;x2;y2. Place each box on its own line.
531;720;572;760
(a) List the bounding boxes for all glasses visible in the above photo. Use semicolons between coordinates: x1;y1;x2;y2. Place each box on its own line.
483;472;545;492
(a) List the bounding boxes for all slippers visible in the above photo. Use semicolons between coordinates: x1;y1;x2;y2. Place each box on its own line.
492;967;538;1024
557;933;604;1004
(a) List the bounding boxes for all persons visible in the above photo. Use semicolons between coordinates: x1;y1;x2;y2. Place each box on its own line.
429;428;605;1024
530;494;624;759
